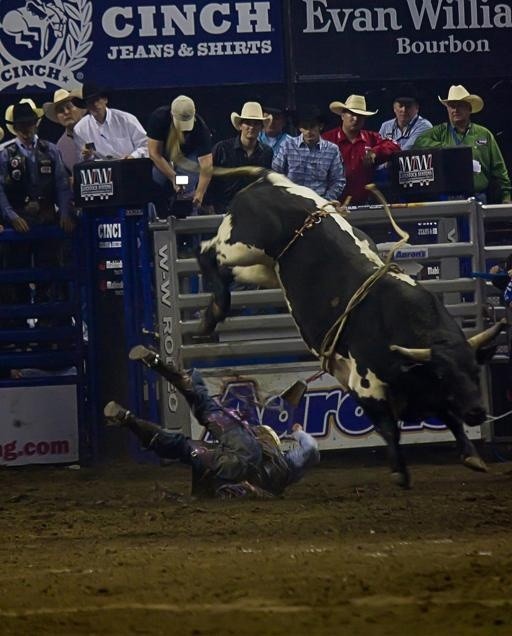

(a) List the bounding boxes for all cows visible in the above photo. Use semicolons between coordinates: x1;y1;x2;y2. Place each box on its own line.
166;122;503;491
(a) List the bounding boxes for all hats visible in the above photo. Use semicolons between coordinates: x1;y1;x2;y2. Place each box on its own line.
171;95;196;131
5;81;113;136
438;85;484;113
231;102;273;132
329;94;379;116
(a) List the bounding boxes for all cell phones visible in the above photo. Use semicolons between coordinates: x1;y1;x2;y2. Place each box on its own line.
86;142;95;151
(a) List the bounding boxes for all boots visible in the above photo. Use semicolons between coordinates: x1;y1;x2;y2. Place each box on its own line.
104;401;130;423
128;345;161;368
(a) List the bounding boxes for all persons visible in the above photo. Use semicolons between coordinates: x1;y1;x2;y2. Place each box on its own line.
104;344;322;502
